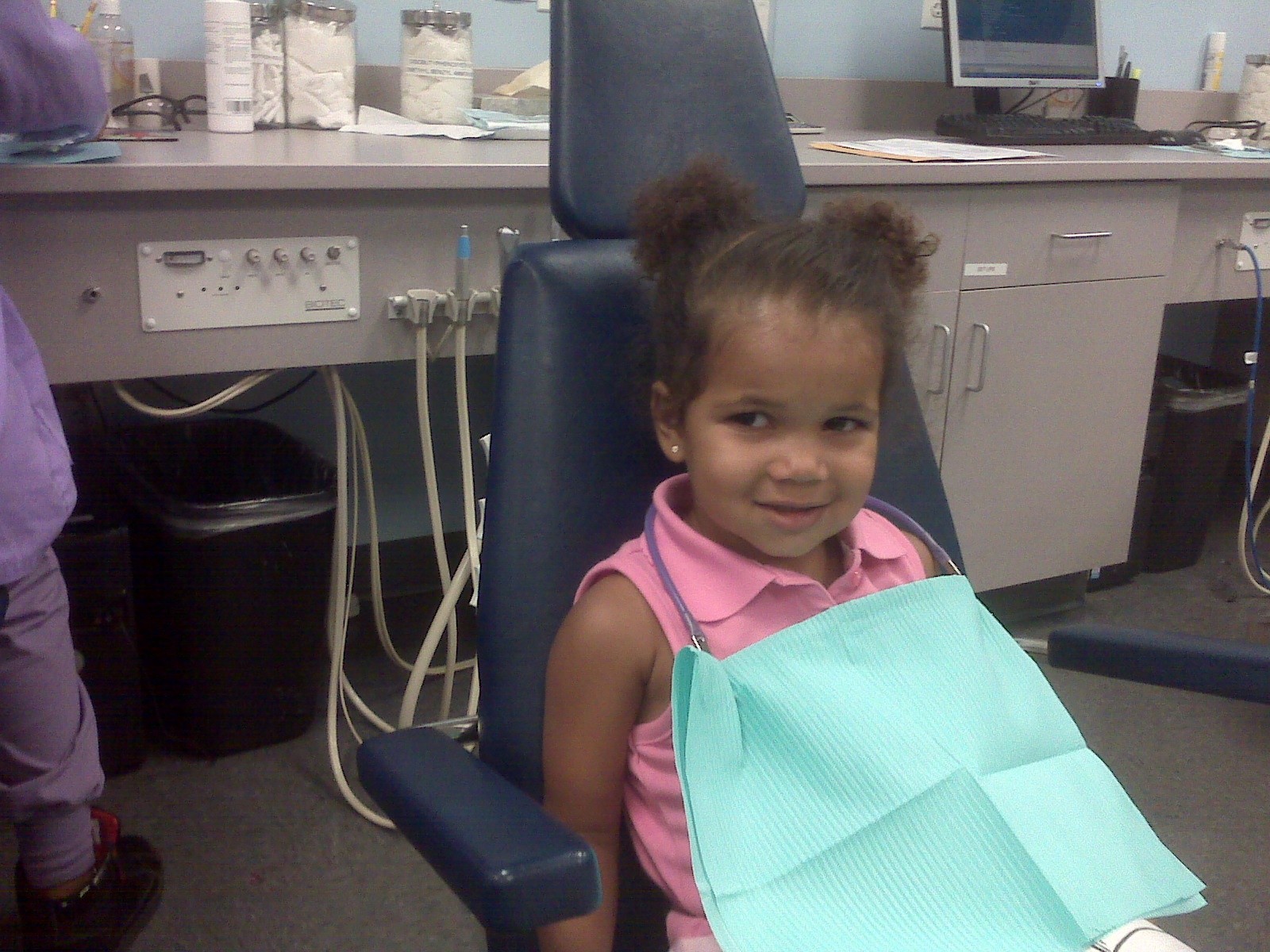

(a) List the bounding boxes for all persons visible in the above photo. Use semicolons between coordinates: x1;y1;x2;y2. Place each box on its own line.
0;0;165;952
538;157;1195;952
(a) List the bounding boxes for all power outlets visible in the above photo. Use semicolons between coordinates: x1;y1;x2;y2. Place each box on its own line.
920;0;944;30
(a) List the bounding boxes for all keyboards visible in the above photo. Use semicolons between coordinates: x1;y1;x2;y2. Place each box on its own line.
934;114;1151;146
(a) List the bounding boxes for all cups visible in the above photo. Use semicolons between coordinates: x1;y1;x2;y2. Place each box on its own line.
1104;77;1139;119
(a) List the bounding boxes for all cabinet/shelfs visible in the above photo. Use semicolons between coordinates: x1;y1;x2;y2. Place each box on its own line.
805;181;1184;621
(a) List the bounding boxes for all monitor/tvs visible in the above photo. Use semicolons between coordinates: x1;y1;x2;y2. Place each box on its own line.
941;0;1107;116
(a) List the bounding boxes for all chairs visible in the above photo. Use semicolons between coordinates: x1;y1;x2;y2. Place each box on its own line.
360;0;1270;952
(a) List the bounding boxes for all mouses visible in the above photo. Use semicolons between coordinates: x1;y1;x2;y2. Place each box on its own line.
1150;129;1206;145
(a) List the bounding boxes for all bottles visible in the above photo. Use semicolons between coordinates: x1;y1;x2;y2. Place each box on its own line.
84;0;134;138
250;3;288;130
280;0;357;131
204;0;256;134
400;8;476;125
